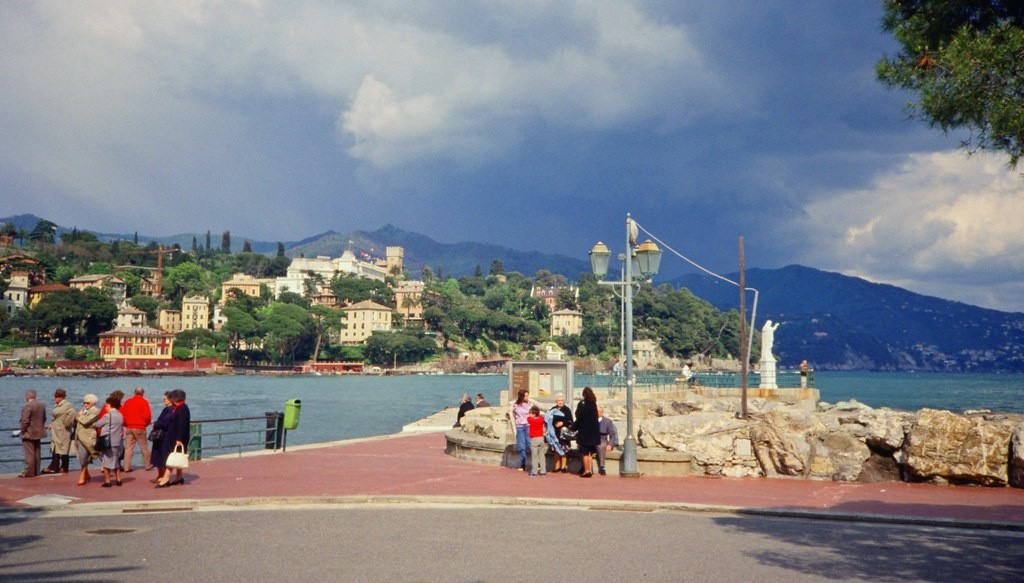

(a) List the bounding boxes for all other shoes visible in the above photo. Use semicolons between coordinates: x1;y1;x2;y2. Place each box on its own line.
101;483;111;487
539;473;546;477
116;481;122;486
528;472;538;477
150;477;161;484
551;467;561;473
18;468;65;478
561;468;568;473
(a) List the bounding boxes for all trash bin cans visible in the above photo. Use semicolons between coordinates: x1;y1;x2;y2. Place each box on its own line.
188;420;202;462
284;396;306;429
266;411;288;451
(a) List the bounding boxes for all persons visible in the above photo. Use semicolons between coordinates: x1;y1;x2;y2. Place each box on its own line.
527;406;546;476
800;360;813;388
17;390;47;477
683;364;699;386
476;394;490;407
75;394;101;485
544;393;573;473
96;397;123;487
123;388;155;473
761;320;779;360
41;389;76;474
506;390;548;471
452;394;474;427
97;390;125;473
149;390;190;488
613;360;620;375
570;387;600;477
597;405;618;476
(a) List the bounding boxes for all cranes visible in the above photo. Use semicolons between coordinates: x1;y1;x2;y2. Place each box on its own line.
125;246;180;294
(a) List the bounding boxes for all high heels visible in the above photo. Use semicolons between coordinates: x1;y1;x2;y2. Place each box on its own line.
155;481;170;488
170;478;184;485
580;472;592;478
77;475;92;486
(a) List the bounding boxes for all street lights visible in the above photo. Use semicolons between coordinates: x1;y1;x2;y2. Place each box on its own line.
588;239;664;477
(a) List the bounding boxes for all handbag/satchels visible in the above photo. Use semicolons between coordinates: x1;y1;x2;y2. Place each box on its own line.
165;444;190;468
93;434;112;451
148;429;163;441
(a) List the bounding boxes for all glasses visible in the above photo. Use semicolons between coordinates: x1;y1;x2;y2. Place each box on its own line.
83;402;92;404
